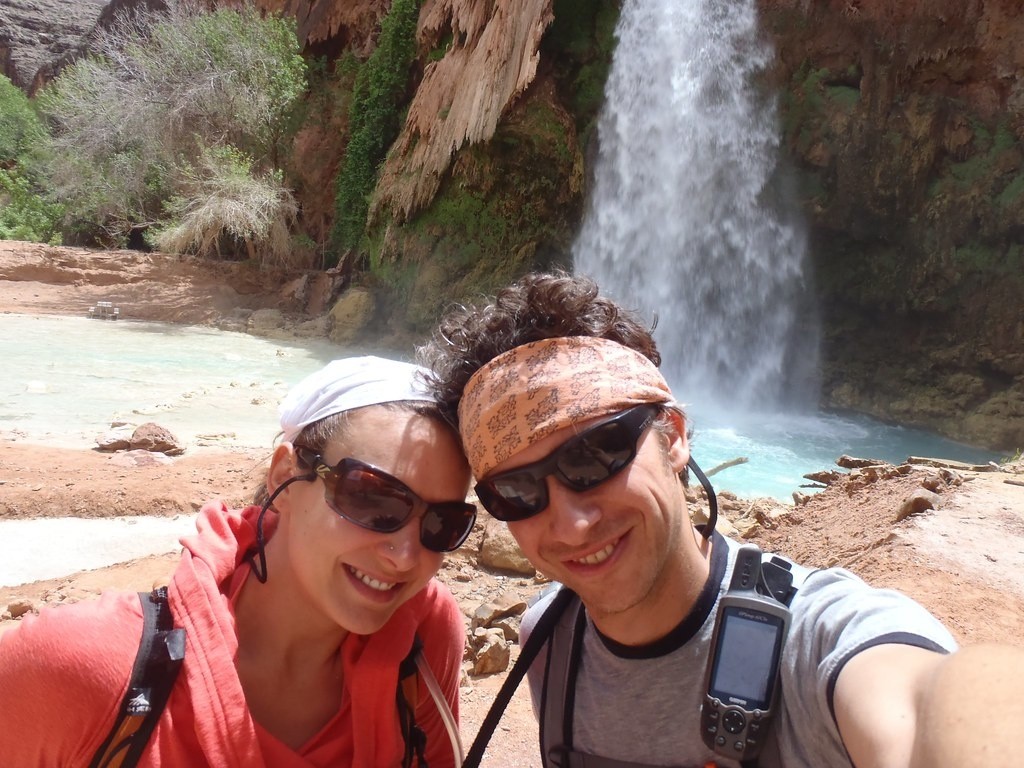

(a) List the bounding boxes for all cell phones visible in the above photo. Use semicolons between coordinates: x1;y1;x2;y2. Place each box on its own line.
700;543;792;762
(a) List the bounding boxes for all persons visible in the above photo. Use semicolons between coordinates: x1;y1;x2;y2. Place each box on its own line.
0;356;473;768
419;272;1024;768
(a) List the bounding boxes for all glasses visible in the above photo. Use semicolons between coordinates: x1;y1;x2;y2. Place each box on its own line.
474;402;660;522
292;444;477;551
